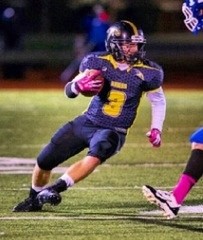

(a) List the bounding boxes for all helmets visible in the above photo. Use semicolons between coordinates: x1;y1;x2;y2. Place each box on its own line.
182;0;203;35
104;19;147;63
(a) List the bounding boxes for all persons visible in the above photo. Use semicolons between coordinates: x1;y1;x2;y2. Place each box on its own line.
11;21;166;213
85;2;114;53
142;0;203;216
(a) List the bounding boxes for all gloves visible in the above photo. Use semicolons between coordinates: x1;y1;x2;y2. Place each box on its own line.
145;128;162;148
74;68;106;97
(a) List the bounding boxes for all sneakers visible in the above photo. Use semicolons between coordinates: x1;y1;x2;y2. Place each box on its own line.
142;184;182;217
12;196;42;212
36;187;68;206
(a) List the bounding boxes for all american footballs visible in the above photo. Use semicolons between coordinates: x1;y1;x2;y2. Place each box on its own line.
78;68;103;98
